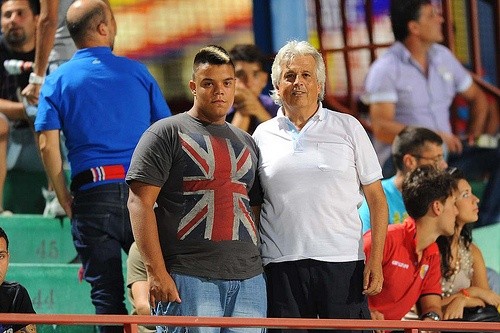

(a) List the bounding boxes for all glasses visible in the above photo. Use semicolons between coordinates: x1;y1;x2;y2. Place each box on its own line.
410;154;445;163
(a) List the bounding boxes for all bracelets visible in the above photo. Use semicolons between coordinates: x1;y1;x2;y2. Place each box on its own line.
29;72;44;84
461;288;470;296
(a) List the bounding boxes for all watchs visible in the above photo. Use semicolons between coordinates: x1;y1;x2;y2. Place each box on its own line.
420;311;440;320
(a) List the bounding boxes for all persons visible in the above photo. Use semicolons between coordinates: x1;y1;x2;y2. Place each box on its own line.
34;0;172;333
127;241;158;333
125;44;267;333
0;0;77;218
225;0;500;333
251;40;388;333
0;226;37;333
